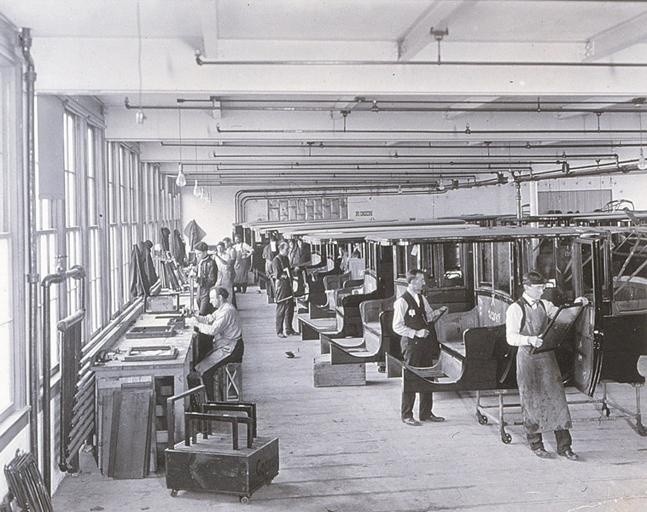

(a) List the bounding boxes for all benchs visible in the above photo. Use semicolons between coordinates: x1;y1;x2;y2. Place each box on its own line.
253;251;515;390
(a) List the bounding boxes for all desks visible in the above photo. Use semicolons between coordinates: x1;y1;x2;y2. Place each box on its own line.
94;291;197;443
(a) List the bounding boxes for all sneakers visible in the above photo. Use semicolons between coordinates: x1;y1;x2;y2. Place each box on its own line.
285;330;299;335
277;332;286;338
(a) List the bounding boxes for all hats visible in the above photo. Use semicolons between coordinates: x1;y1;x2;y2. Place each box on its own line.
193;241;211;251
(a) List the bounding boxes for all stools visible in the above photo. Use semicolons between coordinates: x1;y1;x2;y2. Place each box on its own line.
214;363;242;401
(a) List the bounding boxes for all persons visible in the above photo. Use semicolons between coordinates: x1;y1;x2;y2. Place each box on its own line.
390;267;447;425
260;234;303;340
188;230;256;400
503;270;588;462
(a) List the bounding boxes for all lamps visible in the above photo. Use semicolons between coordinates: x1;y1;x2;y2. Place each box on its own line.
439;163;444;190
135;0;144;124
371;101;378;112
465;123;471;134
175;111;212;204
525;141;531;149
507;142;514;182
562;152;566;158
638;112;647;169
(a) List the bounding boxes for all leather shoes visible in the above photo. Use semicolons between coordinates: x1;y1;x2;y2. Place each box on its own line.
557;447;578;461
531;446;551;458
401;416;420;426
419;413;444;422
